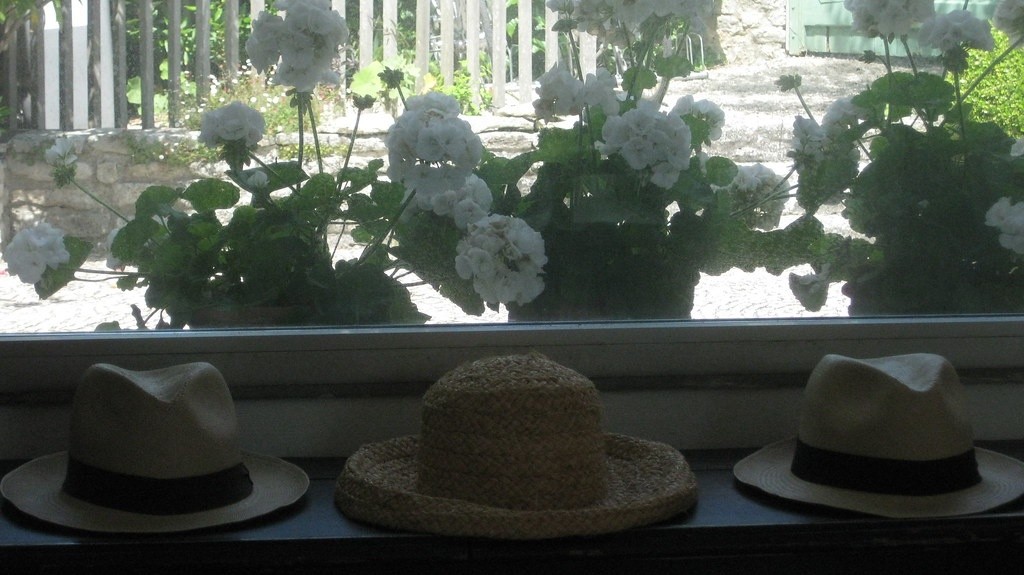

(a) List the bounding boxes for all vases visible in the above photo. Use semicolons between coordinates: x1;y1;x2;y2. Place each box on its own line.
190;304;394;329
509;258;699;325
837;282;1024;317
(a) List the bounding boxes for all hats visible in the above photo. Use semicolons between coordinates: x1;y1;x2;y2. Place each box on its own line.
333;349;700;538
733;351;1024;517
1;359;311;535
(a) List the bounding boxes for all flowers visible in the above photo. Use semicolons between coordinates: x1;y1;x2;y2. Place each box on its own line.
5;0;1024;329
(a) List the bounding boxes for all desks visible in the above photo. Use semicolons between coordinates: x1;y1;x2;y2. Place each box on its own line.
0;437;1024;575
